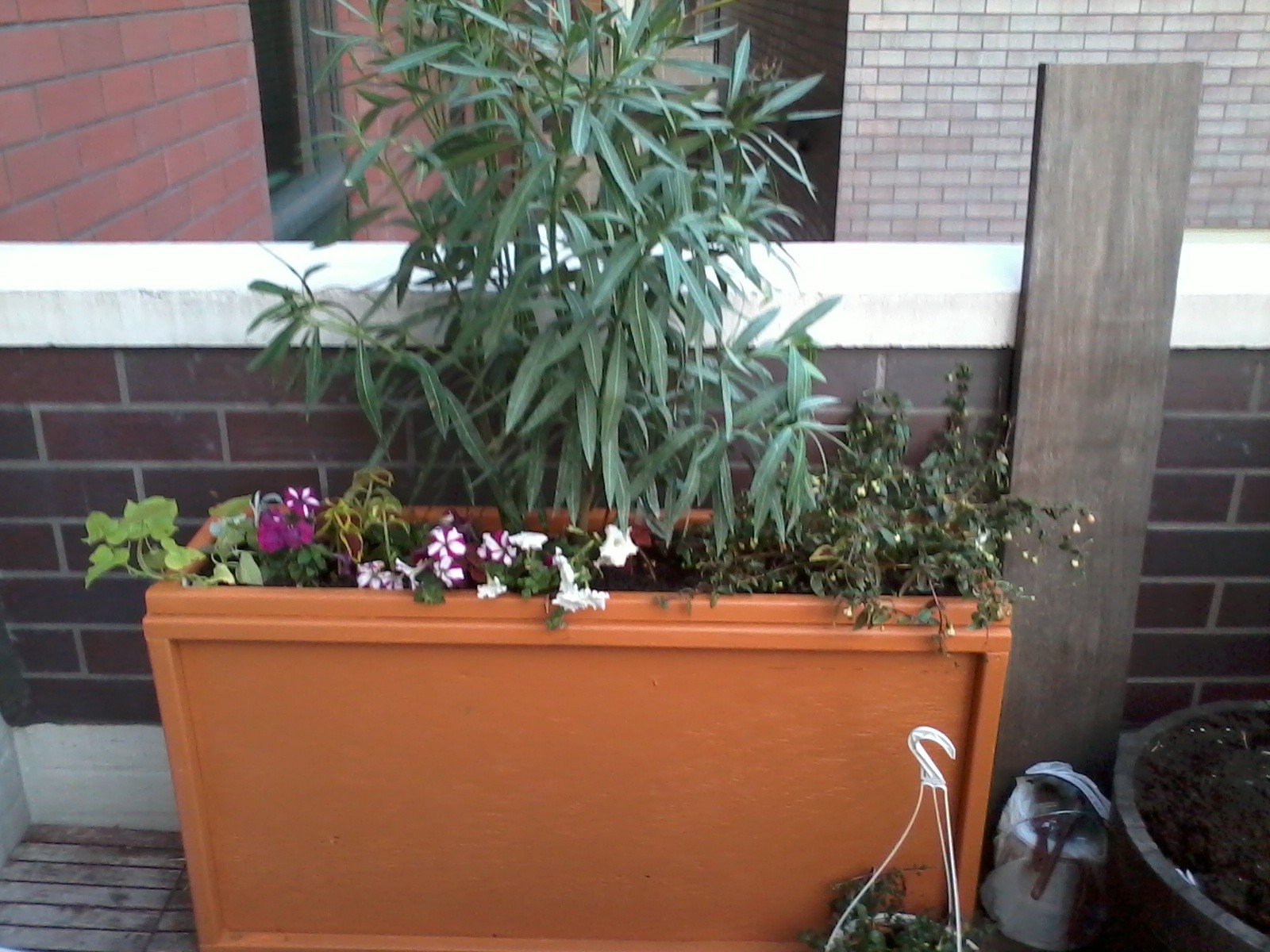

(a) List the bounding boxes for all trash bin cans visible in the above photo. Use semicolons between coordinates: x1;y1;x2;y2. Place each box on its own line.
1;617;32;879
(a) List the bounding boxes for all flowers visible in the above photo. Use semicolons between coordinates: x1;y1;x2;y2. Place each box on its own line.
253;465;639;631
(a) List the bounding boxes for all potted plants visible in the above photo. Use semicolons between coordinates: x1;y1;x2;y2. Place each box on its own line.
801;868;1003;952
78;1;1093;952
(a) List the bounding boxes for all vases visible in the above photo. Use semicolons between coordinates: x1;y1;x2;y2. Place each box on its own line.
1113;700;1270;952
